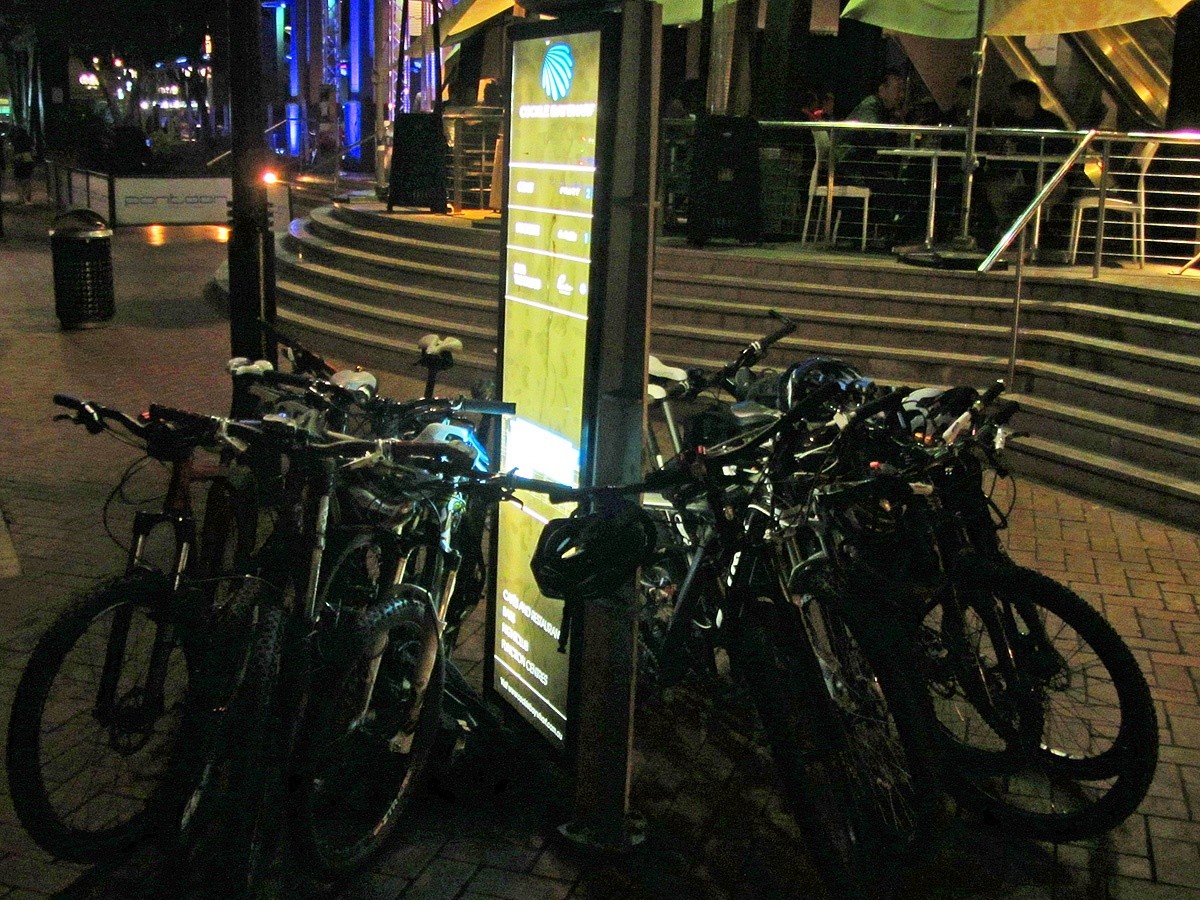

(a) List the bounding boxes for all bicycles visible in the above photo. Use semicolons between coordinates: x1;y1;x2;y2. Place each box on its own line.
548;320;1160;900
4;333;516;880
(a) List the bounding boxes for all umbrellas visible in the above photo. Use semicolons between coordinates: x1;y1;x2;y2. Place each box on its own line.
840;0;1195;253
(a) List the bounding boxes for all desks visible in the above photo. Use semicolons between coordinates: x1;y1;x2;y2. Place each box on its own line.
874;148;986;257
983;154;1099;264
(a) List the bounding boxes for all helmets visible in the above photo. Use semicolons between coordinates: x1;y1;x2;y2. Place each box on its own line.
639;566;673;597
530;499;657;652
776;357;865;413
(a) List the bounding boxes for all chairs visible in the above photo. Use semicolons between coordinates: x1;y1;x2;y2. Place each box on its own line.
800;124;875;251
1067;139;1160;268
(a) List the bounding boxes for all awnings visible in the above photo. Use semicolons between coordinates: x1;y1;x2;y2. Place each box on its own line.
404;0;516;55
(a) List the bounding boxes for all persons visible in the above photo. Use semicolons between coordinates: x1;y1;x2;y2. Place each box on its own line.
0;105;181;204
916;74;990;248
986;79;1077;251
822;70;907;249
663;91;836;232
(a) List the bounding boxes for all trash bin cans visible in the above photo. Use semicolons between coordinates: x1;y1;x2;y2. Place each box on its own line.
49;209;116;328
